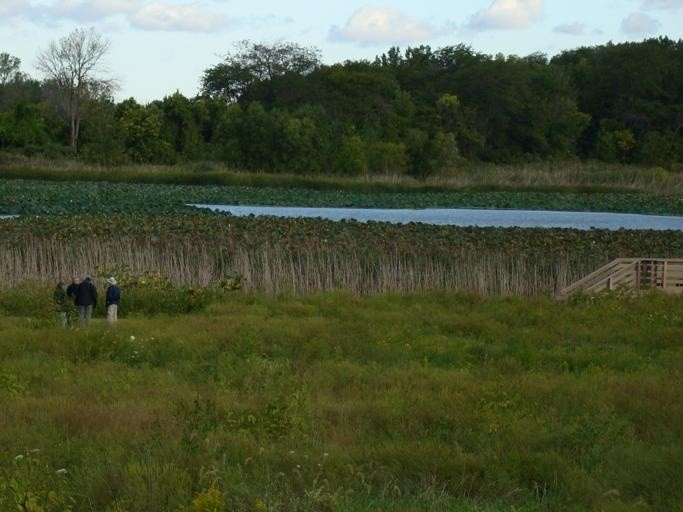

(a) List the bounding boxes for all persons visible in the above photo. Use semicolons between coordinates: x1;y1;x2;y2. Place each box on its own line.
51;281;72;327
74;276;96;328
65;276;79;306
104;276;120;324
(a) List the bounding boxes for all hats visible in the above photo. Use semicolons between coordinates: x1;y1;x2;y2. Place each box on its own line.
106;276;118;286
